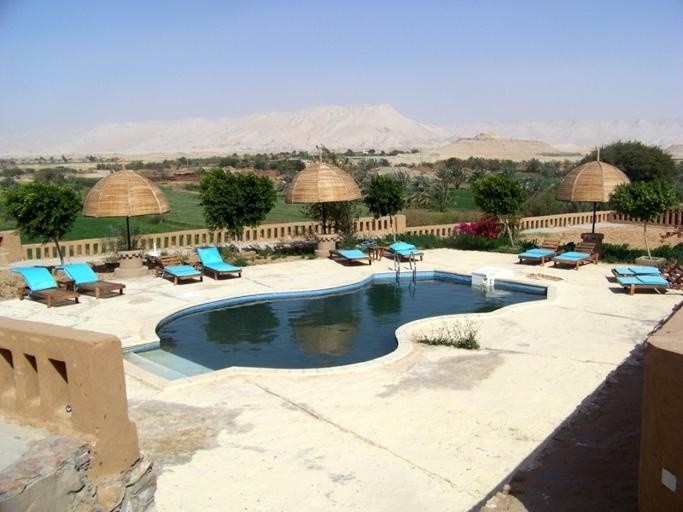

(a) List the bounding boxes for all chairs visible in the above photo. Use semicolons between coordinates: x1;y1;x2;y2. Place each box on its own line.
11;263;125;308
159;247;242;285
611;257;683;295
517;240;599;270
329;242;424;266
